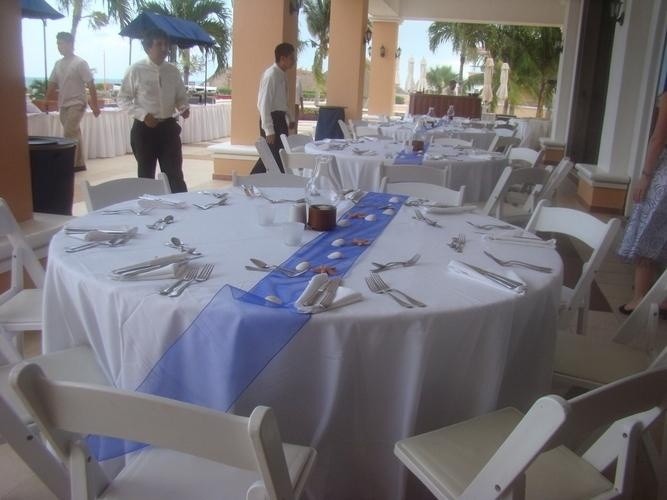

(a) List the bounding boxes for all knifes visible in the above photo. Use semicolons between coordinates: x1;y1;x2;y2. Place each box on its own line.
301;274;345;310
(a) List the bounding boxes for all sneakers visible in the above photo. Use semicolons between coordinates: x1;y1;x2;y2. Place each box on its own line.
73;164;87;172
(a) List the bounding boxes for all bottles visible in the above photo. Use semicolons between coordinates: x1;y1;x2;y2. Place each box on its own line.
290;203;307;228
319;103;490;161
304;152;342;211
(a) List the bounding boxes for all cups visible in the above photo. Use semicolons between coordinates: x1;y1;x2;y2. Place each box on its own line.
280;221;304;247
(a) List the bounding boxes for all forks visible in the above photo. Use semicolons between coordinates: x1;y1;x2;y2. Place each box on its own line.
61;185;275;255
401;194;560;291
161;262;214;301
364;273;426;310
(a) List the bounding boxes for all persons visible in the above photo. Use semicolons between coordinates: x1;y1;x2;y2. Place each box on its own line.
41;31;101;173
616;91;667;319
295;75;304;134
117;29;190;193
250;43;297;175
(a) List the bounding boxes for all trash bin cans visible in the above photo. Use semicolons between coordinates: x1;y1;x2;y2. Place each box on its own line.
315;106;347;141
28;135;79;216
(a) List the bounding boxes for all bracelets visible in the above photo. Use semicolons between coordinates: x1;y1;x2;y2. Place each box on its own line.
643;170;652;177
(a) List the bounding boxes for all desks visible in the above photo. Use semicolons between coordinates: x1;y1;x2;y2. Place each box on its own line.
26;102;233;159
408;93;482;120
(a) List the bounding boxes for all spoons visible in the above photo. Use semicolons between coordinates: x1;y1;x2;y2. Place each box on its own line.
245;258;311;279
364;252;423;271
